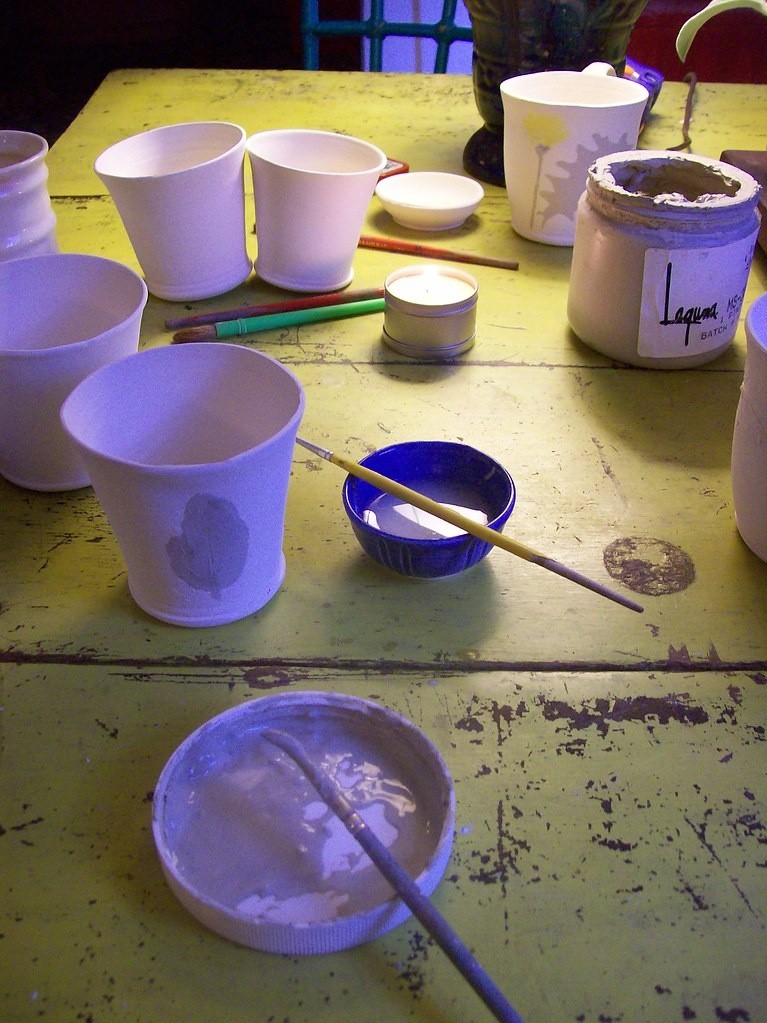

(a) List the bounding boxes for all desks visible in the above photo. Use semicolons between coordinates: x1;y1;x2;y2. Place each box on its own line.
0;68;767;1023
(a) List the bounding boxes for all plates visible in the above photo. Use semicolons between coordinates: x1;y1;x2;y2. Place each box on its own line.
377;173;487;231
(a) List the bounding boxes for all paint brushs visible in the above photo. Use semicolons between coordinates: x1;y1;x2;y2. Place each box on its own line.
290;433;648;615
161;286;389;346
253;724;518;1016
356;234;520;274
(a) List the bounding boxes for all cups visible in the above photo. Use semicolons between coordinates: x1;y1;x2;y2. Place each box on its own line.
93;122;251;300
59;343;306;629
730;293;767;564
1;256;147;492
499;61;649;247
247;130;386;296
1;132;61;268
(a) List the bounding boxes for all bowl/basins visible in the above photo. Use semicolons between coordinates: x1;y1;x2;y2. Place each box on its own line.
342;442;516;578
